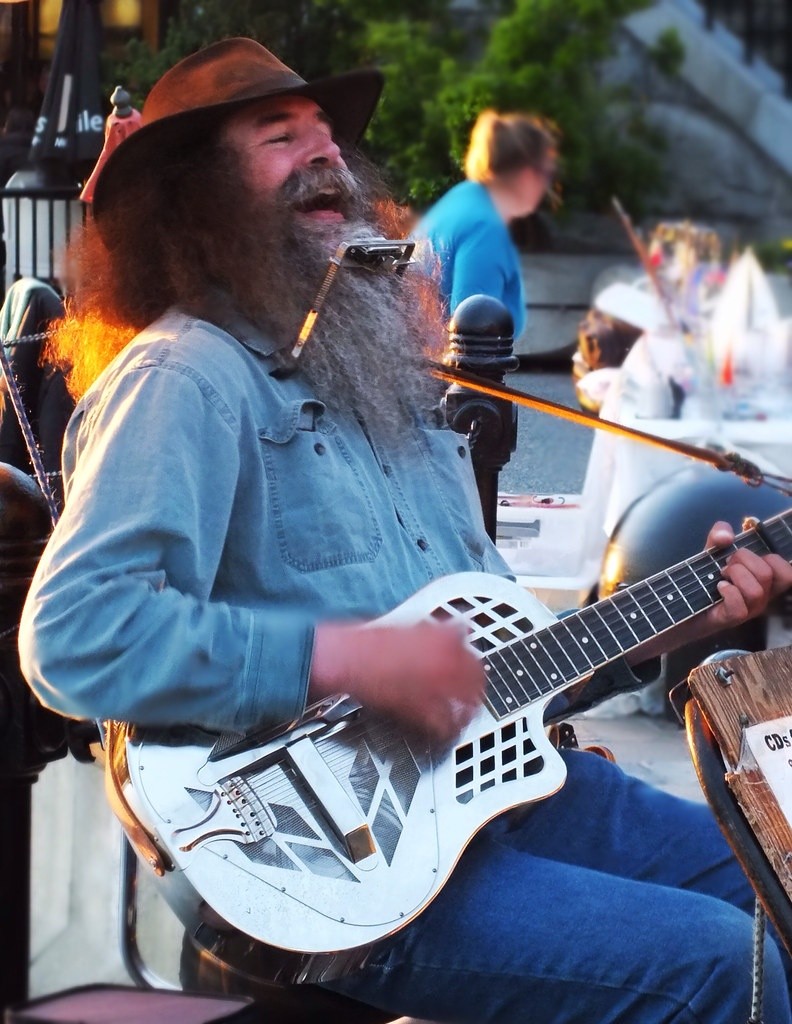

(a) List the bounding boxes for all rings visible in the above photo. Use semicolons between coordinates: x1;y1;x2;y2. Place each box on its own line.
450;699;463;722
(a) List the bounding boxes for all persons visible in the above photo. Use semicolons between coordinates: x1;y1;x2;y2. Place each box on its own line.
400;109;555;374
19;37;792;1024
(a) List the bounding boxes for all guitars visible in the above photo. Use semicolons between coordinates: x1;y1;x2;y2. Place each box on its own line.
101;498;790;991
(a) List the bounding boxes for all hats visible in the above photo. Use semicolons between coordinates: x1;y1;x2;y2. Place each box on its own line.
92;38;385;267
